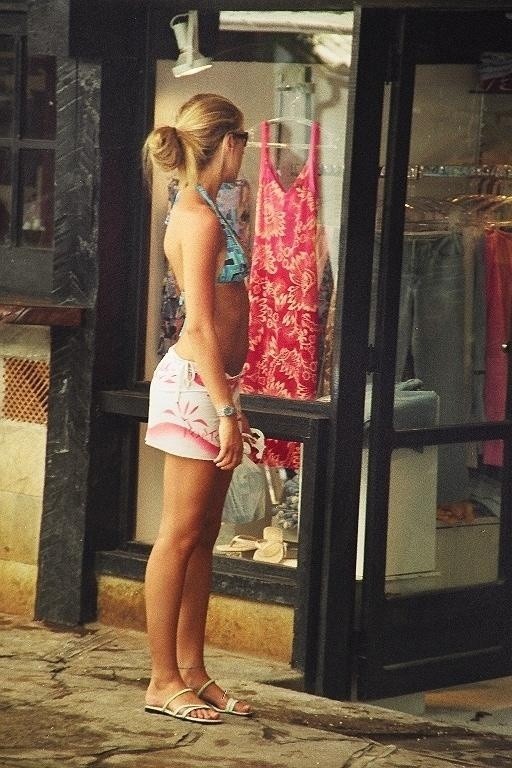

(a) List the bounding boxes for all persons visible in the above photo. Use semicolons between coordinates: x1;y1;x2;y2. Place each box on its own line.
143;94;255;725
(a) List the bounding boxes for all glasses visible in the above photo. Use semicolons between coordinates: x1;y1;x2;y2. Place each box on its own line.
234;131;249;147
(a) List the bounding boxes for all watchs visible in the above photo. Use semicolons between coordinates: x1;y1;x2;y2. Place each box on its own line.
217;406;237;416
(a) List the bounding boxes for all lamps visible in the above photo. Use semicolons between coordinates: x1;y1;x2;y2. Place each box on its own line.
167;9;214;79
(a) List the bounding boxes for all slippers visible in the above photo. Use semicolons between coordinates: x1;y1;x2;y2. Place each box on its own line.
198;677;255;716
144;687;223;724
219;526;285;564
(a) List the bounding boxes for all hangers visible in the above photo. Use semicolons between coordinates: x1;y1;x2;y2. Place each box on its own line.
405;169;512;237
245;85;339;149
